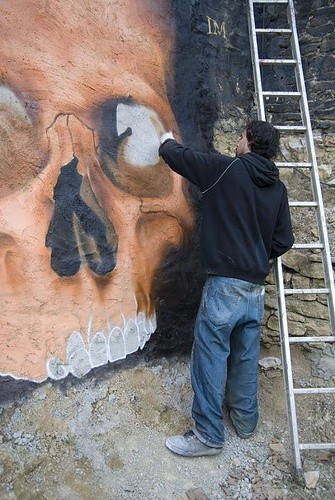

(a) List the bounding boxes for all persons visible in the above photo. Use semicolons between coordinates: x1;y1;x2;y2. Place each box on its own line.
157;117;296;458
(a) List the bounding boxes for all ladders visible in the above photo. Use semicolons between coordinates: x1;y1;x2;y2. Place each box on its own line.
246;0;334;485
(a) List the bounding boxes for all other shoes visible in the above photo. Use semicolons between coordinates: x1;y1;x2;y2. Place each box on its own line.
165;430;223;456
